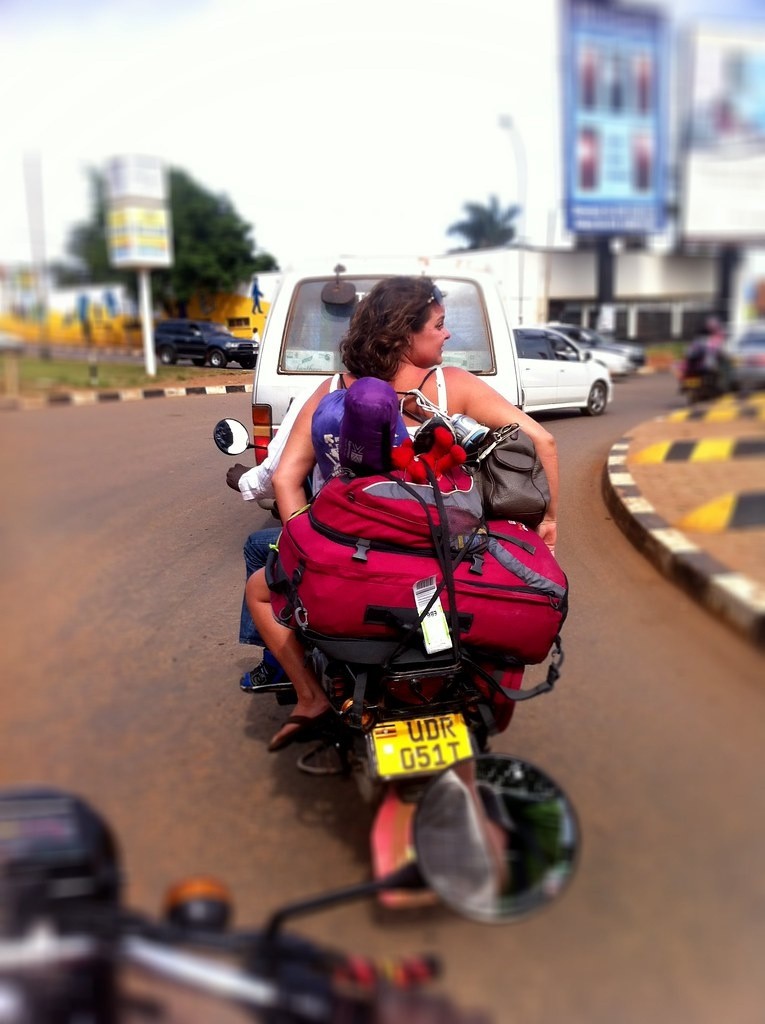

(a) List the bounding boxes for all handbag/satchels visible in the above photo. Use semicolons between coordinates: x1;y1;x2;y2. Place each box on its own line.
459;422;552;530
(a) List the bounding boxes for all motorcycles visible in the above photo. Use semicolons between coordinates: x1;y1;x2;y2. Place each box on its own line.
213;416;491;911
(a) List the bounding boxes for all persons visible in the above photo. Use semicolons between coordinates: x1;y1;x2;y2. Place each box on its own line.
245;276;558;753
251;328;260;344
226;379;325;690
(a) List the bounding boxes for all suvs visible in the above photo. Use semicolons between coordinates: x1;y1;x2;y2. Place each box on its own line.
155;320;259;370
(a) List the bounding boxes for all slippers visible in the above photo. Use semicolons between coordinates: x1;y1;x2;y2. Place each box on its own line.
268;705;330;754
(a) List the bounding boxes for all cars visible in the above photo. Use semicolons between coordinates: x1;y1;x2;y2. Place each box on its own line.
547;324;647;366
725;322;765;392
513;328;615;417
557;342;638;374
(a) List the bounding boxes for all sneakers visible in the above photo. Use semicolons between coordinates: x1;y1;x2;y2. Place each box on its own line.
413;413;491;455
240;647;296;694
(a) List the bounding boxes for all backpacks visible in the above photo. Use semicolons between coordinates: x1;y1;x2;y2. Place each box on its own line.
262;451;568;703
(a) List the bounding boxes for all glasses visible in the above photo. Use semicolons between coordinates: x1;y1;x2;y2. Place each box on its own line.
407;286;443;322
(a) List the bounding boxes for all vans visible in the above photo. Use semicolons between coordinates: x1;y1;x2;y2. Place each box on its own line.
250;261;527;518
